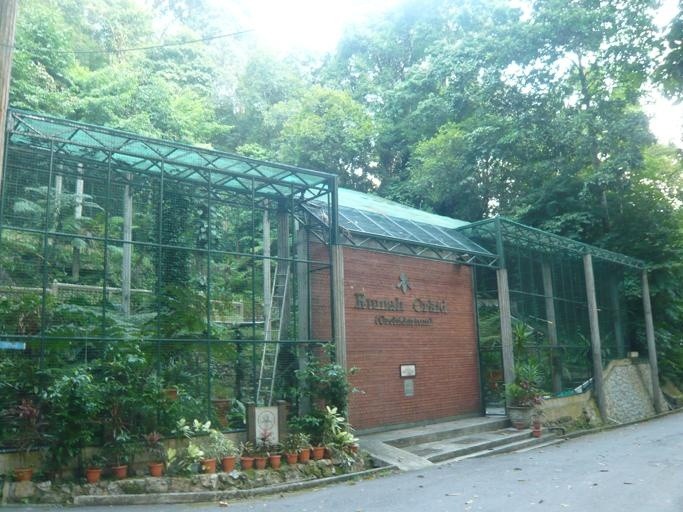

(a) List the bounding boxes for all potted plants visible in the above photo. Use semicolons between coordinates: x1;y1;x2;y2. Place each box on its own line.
1;395;360;483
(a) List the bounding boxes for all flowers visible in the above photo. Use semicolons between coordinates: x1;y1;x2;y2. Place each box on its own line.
506;358;550;407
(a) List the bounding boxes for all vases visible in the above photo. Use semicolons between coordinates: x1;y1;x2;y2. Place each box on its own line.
506;404;534;429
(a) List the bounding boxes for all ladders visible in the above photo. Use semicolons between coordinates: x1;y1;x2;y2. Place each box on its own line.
255;261;291;408
